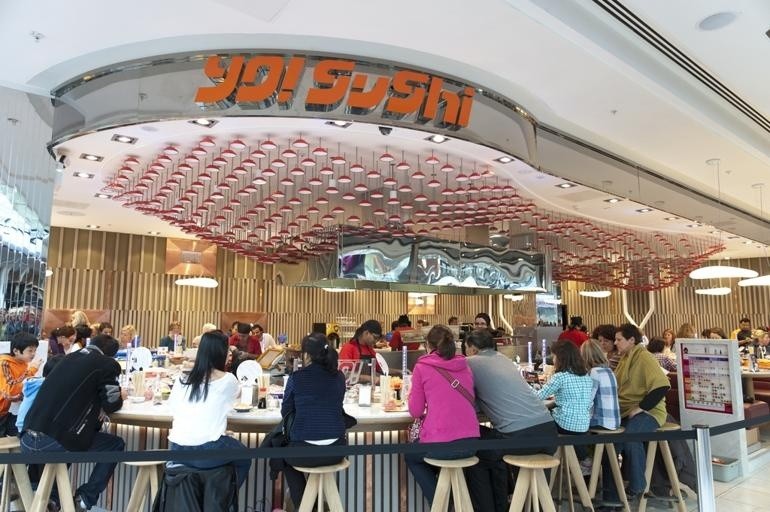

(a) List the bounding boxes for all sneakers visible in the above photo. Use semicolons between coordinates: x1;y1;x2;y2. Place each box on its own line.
625;489;635;502
594;505;621;512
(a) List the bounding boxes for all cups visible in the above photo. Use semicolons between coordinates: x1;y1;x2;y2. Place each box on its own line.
358;385;371;407
380;374;392;407
401;374;412;403
265;394;278;411
241;387;253;405
384;390;397;410
258;373;270;408
282;375;289;392
132;370;145;402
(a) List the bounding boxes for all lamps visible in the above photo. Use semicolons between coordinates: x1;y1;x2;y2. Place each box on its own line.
531;211;725;292
100;133;535;265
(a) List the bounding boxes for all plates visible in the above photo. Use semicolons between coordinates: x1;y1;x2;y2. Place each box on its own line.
237;358;263;386
234;405;252;411
375;352;389;376
131;346;152;371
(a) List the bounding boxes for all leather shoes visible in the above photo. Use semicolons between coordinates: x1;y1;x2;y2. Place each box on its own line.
46;502;58;512
73;495;87;512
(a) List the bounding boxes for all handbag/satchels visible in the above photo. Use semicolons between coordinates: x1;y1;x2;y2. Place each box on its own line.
407;367;477;443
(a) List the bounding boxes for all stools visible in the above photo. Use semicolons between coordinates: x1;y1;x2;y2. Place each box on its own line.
122;449;166;512
588;426;631;512
549;434;595;512
30;463;75;512
638;422;686;512
425;459;479;511
506;453;560;512
0;437;35;512
291;459;351;512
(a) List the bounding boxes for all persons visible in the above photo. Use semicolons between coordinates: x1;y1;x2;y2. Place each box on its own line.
0;334;44;438
119;325;137;349
99;322;113;336
756;332;770;359
251;325;275;352
731;318;759;354
677;323;696;338
581;324;591;338
64;327;85;355
416;320;426;350
167;329;253;492
581;339;621;511
47;326;76;359
612;324;671;499
449;316;458;326
226;322;241;337
663;330;677;356
403;325;480;511
465;329;557;511
339;319;412;387
639;328;649;347
19;334;126;512
374;339;387;348
388;314;421;351
384;322;399;342
707;327;727;339
280;333;346;512
461;312;498;356
229;323;261;378
193;323;217;348
647;337;678;372
70;310;93;342
536;339;593;469
160;322;186;351
593;324;625;372
557;316;589;348
